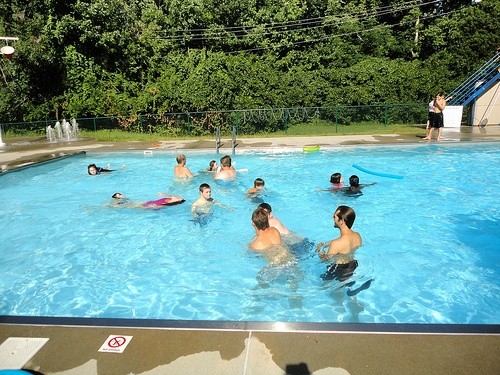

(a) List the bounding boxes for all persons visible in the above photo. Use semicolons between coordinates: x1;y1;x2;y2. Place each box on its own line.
102;193;125;208
246;207;302;290
214;155;237;182
174;154;193;179
326;173;344;191
206;160;222;173
256;202;316;259
190;183;237;220
118;193;186;210
245;177;264;198
422;92;445;141
316;205;363;281
318;174;377;199
87;163;126;176
